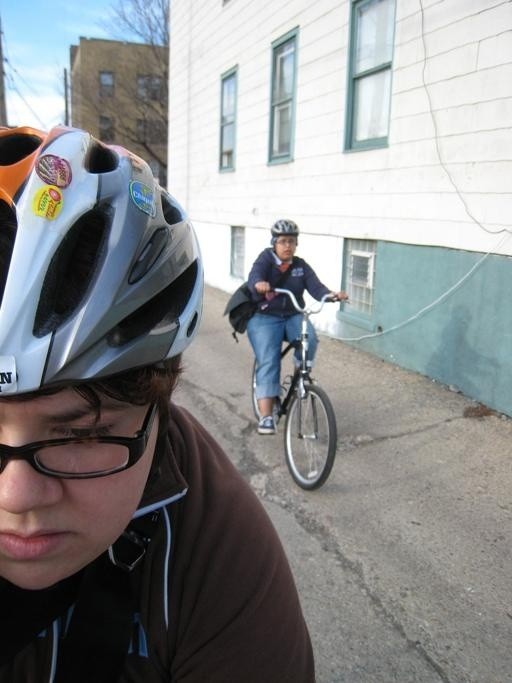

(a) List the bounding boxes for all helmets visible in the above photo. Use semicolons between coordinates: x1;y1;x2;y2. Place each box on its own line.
1;123;205;398
272;219;299;235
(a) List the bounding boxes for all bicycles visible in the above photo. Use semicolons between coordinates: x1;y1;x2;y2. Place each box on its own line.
251;288;338;490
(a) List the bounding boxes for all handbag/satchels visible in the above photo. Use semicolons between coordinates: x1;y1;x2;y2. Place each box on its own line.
222;281;257;335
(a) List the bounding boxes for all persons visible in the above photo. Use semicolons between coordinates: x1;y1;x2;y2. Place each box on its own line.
223;220;348;435
0;126;315;683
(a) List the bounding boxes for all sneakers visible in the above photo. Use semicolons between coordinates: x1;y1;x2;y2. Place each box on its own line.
259;414;277;436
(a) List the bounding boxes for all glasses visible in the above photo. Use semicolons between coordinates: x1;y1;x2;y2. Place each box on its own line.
277;239;296;244
0;400;175;477
